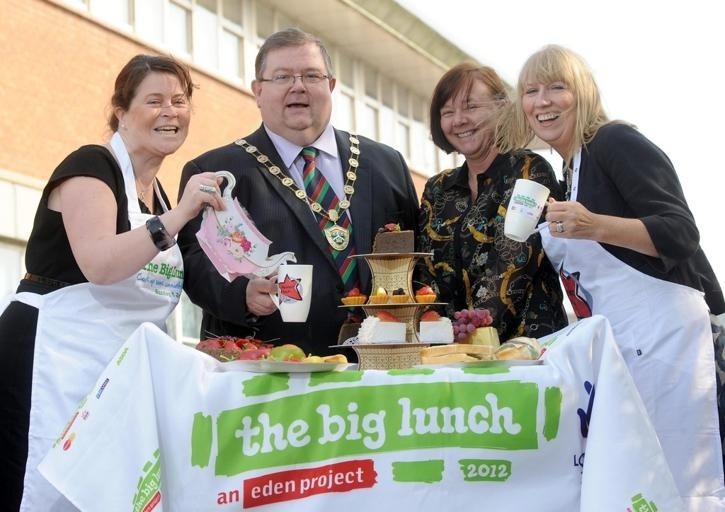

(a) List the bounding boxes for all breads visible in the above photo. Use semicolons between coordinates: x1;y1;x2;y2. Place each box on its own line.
419;344;493;364
495;336;540;360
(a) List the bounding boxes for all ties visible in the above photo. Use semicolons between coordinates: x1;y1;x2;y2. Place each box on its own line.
299;145;361;314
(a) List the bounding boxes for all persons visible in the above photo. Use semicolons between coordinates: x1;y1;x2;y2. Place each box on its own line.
170;28;423;359
406;62;571;348
0;52;230;510
490;42;724;512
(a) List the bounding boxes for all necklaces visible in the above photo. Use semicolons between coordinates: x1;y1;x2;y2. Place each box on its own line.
234;128;361;252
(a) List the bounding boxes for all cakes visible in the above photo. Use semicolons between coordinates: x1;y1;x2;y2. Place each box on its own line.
419;310;455;343
460;325;500;348
372;223;414;253
341;285;437;305
357;311;407;345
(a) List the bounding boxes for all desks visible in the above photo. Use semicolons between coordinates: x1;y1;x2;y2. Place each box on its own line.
142;317;634;511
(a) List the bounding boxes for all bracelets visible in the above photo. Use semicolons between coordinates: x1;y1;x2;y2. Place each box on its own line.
145;216;177;254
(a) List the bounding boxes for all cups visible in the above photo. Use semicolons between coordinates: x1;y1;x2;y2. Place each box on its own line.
269;264;314;323
504;179;558;243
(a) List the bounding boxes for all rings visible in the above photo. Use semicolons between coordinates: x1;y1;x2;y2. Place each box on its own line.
555;223;565;234
199;184;216;193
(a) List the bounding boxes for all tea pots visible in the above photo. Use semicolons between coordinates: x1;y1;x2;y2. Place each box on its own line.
195;170;299;284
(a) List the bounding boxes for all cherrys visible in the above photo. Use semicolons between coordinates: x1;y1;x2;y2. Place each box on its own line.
196;330;281;351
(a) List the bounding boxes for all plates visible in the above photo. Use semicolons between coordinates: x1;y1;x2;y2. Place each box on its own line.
415;359;545;370
218;359;339;373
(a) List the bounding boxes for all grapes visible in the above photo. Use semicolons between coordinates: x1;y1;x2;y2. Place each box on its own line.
452;308;493;341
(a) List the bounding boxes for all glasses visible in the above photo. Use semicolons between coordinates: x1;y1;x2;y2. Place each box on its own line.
257;72;331;85
